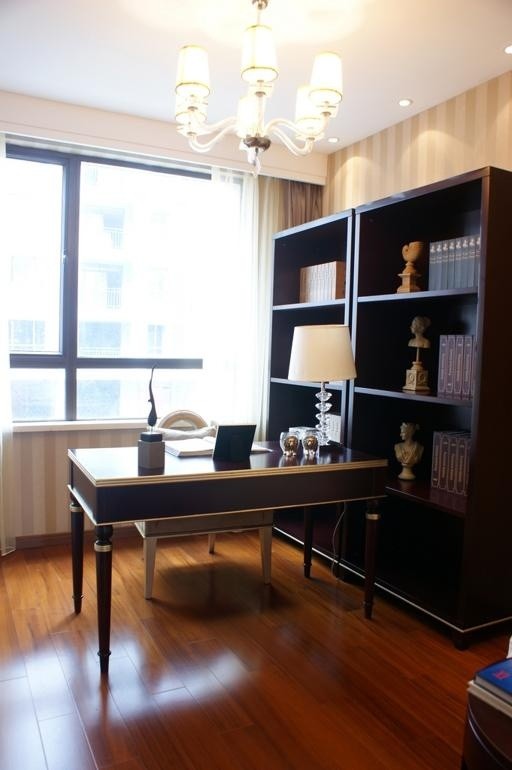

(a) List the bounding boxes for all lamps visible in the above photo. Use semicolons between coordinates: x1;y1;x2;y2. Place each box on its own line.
288;323;355;454
173;0;344;177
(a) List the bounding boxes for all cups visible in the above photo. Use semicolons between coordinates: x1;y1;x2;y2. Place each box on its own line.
279;430;301;456
302;429;322;458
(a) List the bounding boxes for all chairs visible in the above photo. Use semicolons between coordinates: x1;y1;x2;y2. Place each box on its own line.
135;409;274;599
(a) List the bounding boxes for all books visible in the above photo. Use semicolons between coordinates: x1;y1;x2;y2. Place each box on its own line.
324;411;342;444
426;427;475;493
426;236;480;290
294;260;348;303
466;655;512;721
434;335;477;401
162;433;274;458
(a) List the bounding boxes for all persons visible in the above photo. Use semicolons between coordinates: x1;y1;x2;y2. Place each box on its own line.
393;422;424;481
407;317;431;348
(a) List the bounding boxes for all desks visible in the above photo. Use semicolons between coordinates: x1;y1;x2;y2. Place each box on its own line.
65;441;390;677
458;682;512;769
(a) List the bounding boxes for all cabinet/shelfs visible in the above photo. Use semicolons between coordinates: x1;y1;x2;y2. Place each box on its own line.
262;210;353;579
352;166;511;648
